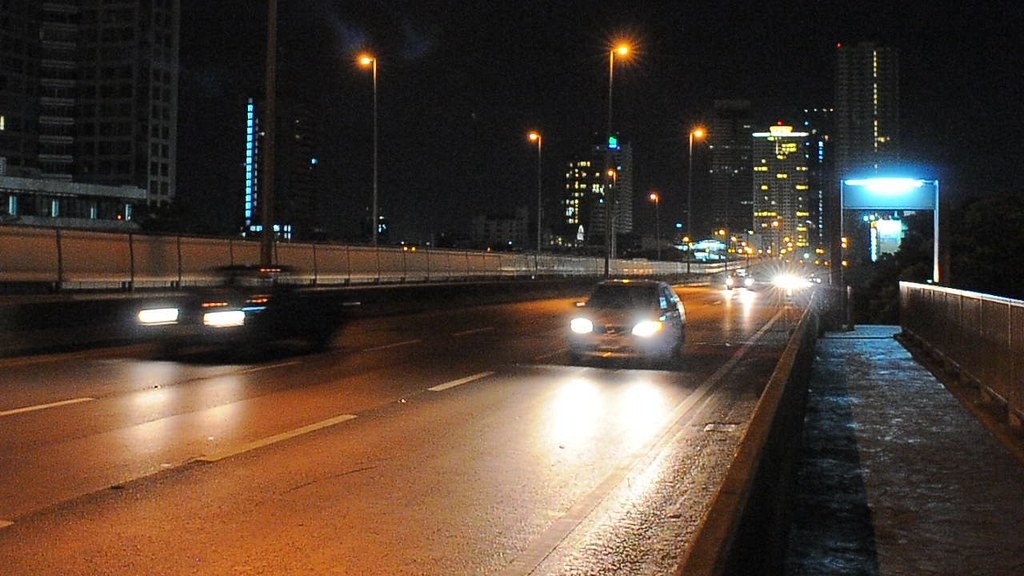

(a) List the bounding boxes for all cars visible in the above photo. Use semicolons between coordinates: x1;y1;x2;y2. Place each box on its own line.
567;278;688;367
131;260;348;354
725;253;822;292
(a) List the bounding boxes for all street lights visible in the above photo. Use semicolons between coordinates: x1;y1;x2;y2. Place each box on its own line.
528;131;542;254
685;130;703;272
651;193;662;261
718;222;780;268
601;43;628;278
358;55;381;280
608;169;619;259
